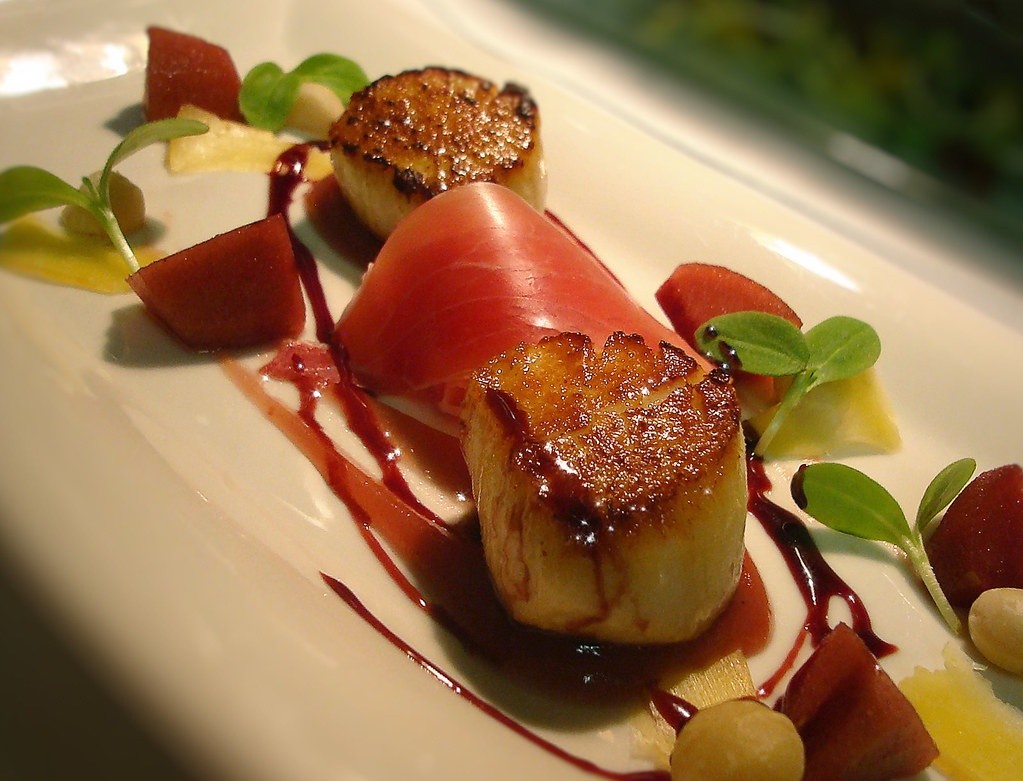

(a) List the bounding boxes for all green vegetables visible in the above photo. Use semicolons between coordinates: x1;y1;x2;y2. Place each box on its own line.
0;53;374;276
698;311;976;634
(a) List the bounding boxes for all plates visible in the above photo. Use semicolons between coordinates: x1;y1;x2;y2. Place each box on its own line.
0;1;1022;781
